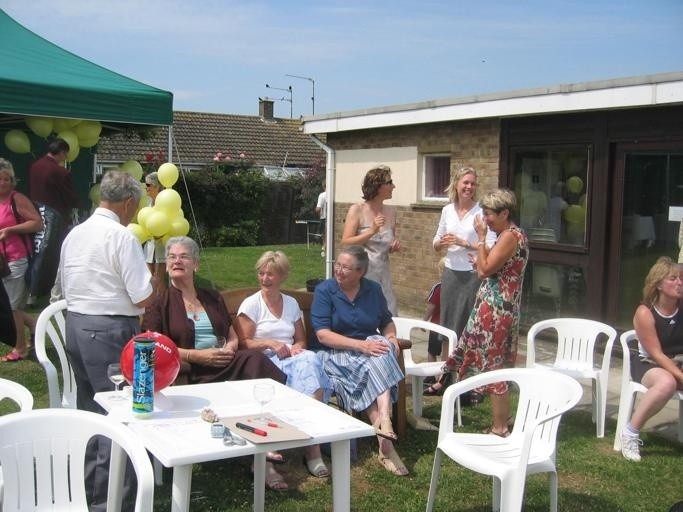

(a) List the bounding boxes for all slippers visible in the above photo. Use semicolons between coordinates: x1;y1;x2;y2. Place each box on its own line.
0;351;29;362
27;333;35;351
302;454;330;478
266;452;287;464
249;463;288;490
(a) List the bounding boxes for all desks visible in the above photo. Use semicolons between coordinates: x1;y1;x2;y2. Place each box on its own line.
92;375;376;512
299;219;321;255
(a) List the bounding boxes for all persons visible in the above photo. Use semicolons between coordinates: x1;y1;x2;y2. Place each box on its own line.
340;165;401;317
0;157;44;364
619;255;682;464
438;190;530;439
57;171;159;510
418;256;445;364
545;178;568;242
313;178;326;257
139;237;287;494
234;251;334;479
139;168;175;340
26;138;82;307
423;165;497;406
309;243;410;477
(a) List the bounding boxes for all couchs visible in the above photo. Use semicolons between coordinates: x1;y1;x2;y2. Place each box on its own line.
220;287;411;432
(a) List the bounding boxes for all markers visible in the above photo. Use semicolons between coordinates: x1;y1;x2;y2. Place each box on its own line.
236;422;267;436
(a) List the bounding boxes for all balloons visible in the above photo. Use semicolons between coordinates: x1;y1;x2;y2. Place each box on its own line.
156;163;179;188
154;189;181;215
127;223;141;238
129;183;147;224
578;194;587;207
74;121;101;140
78;138;99;148
170;219;189;236
25;117;53;138
53;118;101;148
66;148;79;162
51;132;80;166
563;204;584;225
178;209;183;223
563;174;581;196
4;130;30;154
119;330;180;392
136;207;154;224
53;119;71;134
88;184;103;203
123;160;142;182
145;212;170;237
565;175;583;195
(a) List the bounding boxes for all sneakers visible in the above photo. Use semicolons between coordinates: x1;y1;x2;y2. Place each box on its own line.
619;427;641;463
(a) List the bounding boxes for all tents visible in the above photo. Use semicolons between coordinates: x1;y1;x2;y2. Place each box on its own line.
0;7;173;164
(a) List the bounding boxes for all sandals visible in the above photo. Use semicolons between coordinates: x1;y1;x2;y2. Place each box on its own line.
470;391;484;404
377;449;410;477
486;427;509;438
423;377;436;384
372;417;399;441
490;415;514;430
423;383;448;396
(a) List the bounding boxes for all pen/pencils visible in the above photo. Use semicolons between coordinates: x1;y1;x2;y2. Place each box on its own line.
248;419;281;428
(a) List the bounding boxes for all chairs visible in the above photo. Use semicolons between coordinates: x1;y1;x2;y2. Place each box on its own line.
529;228;562;318
33;298;79;410
0;406;155;512
421;365;584;511
613;330;683;455
387;316;464;429
524;316;617;441
0;377;34;500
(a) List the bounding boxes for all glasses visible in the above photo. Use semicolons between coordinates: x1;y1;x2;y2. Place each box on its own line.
145;183;154;187
334;263;355;272
167;255;189;263
461;167;469;171
384;180;393;186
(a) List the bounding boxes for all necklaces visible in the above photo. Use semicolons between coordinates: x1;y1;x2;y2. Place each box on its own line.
182;295;200;322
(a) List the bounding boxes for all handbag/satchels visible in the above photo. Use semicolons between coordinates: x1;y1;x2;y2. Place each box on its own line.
406;411;457;431
11;193;64;296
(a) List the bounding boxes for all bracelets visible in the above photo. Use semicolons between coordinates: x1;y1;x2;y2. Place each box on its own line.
387;336;398;342
474;241;490;249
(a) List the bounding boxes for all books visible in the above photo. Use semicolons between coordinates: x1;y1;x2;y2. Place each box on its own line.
217;413;314;445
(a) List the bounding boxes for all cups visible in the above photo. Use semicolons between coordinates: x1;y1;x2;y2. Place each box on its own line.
254;382;273;412
108;364;122;396
